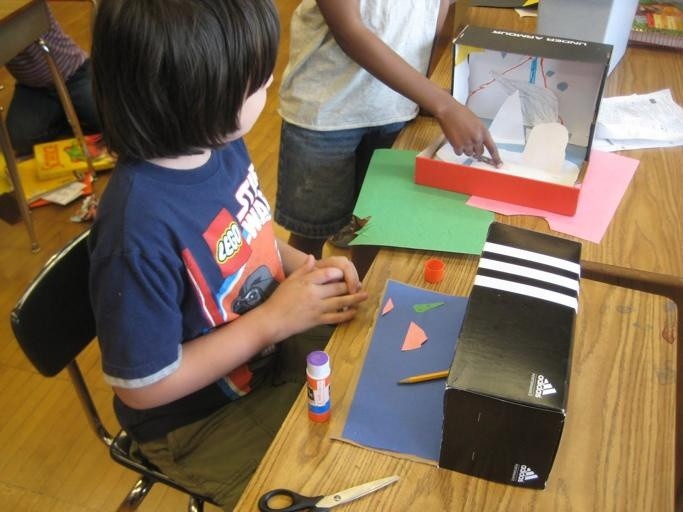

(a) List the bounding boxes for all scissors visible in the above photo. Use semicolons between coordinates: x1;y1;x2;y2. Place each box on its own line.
258;475;398;512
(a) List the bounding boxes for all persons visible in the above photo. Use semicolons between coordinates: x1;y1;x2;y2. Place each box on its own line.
0;3;102;156
272;0;504;282
87;0;372;509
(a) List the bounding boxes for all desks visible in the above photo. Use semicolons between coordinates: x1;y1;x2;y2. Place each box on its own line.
372;1;681;287
232;277;681;512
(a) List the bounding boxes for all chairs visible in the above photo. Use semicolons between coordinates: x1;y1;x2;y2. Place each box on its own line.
11;219;214;512
3;1;98;254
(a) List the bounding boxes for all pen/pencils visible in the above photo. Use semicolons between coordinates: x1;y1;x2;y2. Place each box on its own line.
398;369;450;384
